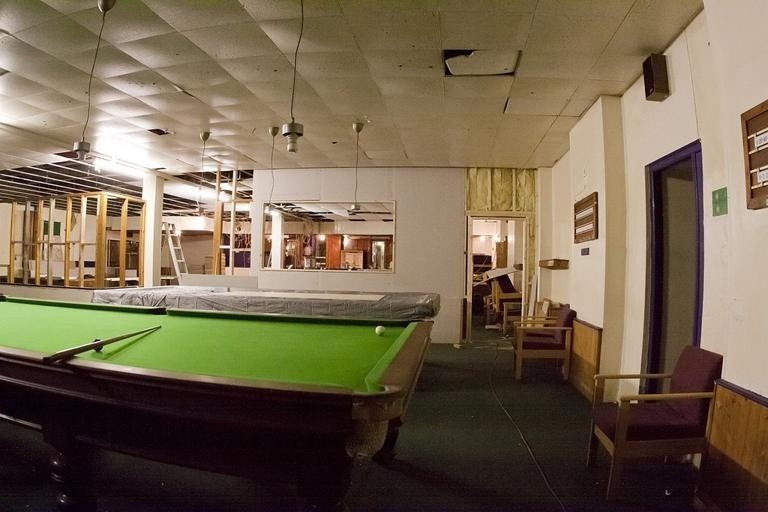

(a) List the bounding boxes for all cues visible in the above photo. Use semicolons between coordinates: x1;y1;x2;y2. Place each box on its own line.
43;326;161;363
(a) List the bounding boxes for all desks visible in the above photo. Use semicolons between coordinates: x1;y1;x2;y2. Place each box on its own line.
0;284;441;512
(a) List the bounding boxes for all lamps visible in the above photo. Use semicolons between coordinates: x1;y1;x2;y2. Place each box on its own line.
280;1;305;153
265;127;278;216
351;122;361;210
72;0;116;161
195;132;211;210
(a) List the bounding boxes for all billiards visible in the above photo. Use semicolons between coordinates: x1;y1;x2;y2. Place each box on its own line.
93;339;103;351
375;326;385;335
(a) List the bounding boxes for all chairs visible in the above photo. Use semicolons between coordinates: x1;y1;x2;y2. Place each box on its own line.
483;281;576;380
587;345;724;503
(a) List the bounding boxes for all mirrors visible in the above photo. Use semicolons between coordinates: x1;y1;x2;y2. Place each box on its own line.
260;200;397;274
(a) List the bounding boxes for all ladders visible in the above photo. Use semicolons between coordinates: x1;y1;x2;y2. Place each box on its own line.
216;170;238;276
161;222;189;285
